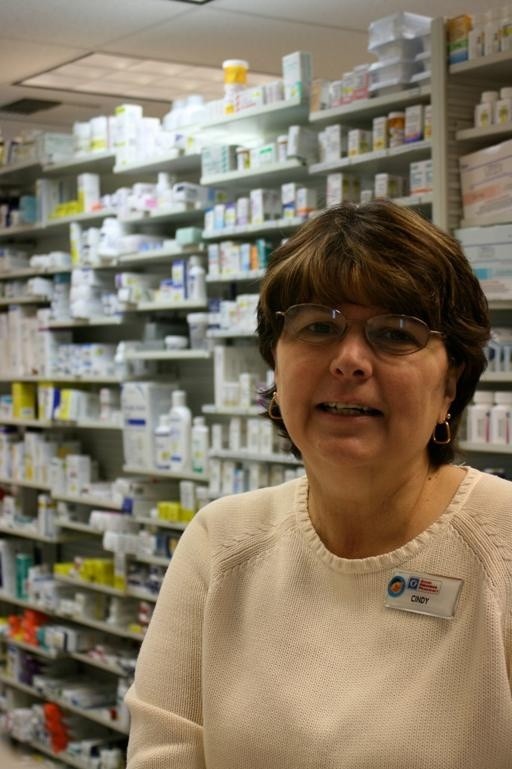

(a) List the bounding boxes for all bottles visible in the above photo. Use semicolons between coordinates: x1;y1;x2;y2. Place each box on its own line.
186;255;206;301
466;3;511;124
149;390;276;529
465;389;512;446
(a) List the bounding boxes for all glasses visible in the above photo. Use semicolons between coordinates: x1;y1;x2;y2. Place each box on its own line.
276;303;446;356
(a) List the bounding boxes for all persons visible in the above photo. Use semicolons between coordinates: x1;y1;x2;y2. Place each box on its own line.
123;197;512;768
